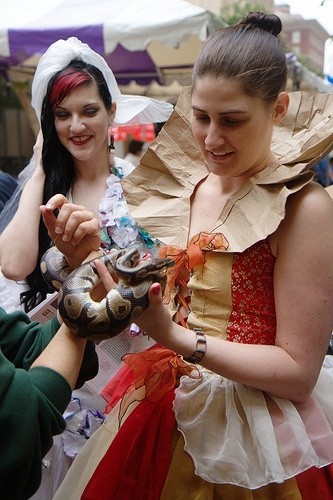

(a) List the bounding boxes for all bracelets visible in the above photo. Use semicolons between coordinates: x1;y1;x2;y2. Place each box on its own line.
186;330;209;363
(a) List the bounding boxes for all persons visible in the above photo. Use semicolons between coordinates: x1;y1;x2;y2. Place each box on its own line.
92;11;332;500
0;36;159;373
0;193;176;500
